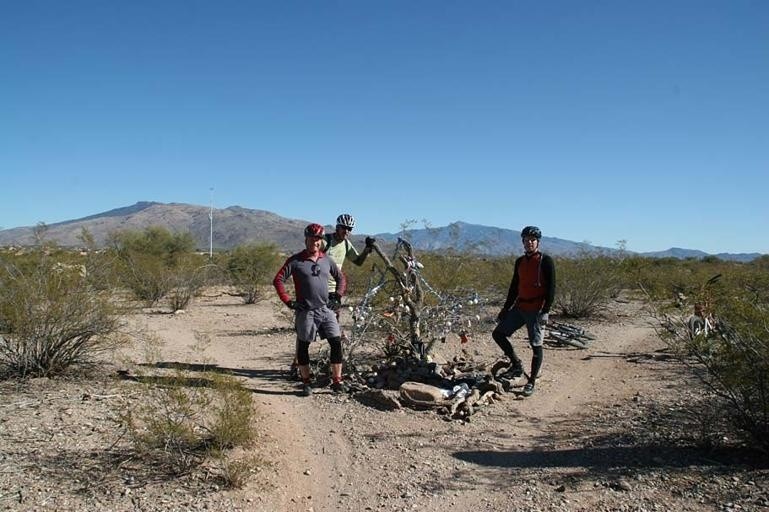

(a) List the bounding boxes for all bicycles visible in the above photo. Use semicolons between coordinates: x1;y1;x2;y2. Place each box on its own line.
541;320;596;349
670;274;723;347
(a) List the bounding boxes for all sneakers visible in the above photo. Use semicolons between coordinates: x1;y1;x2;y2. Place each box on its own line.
523;378;535;396
303;382;312;395
501;360;523;379
333;382;344;392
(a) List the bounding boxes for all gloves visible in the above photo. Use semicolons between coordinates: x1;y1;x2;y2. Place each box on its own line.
366;236;376;249
538;313;549;324
288;301;305;312
498;308;509;319
327;298;341;310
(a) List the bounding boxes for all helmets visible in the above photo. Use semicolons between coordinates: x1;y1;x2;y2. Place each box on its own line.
337;214;355;228
305;224;325;238
521;226;542;238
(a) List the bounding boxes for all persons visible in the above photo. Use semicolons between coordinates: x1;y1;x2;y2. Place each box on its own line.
272;222;354;398
493;226;555;397
290;212;376;382
